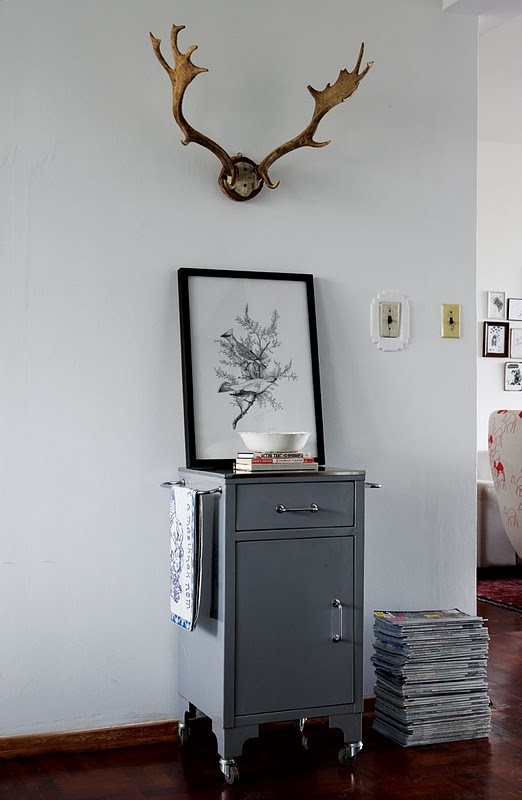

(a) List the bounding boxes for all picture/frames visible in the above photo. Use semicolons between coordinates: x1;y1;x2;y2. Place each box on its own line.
177;267;326;472
487;289;506;321
506;297;522;322
504;362;522;392
509;327;522;360
483;321;509;359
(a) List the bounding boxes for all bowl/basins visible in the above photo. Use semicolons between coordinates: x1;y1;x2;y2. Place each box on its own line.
238;430;313;453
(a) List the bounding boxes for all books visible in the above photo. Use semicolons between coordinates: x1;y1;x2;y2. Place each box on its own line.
370;608;493;749
233;451;318;473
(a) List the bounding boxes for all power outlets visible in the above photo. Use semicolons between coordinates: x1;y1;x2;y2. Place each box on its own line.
441;303;461;338
379;301;401;338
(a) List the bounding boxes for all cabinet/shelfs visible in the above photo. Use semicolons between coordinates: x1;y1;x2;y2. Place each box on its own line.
160;467;382;783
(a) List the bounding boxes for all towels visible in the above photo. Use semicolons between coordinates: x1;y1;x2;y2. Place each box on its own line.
168;485;204;631
